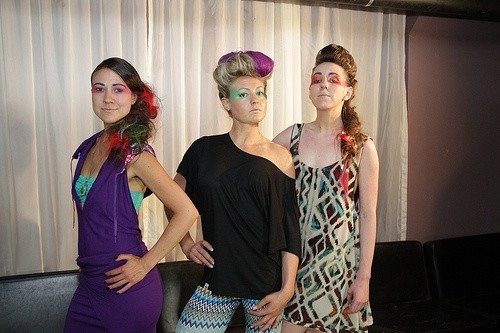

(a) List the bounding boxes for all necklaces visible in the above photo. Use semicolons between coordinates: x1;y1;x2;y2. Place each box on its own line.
81;145;112;196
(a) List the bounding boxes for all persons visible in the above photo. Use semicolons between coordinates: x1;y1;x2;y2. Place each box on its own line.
63;58;200;333
165;51;299;333
272;44;379;333
